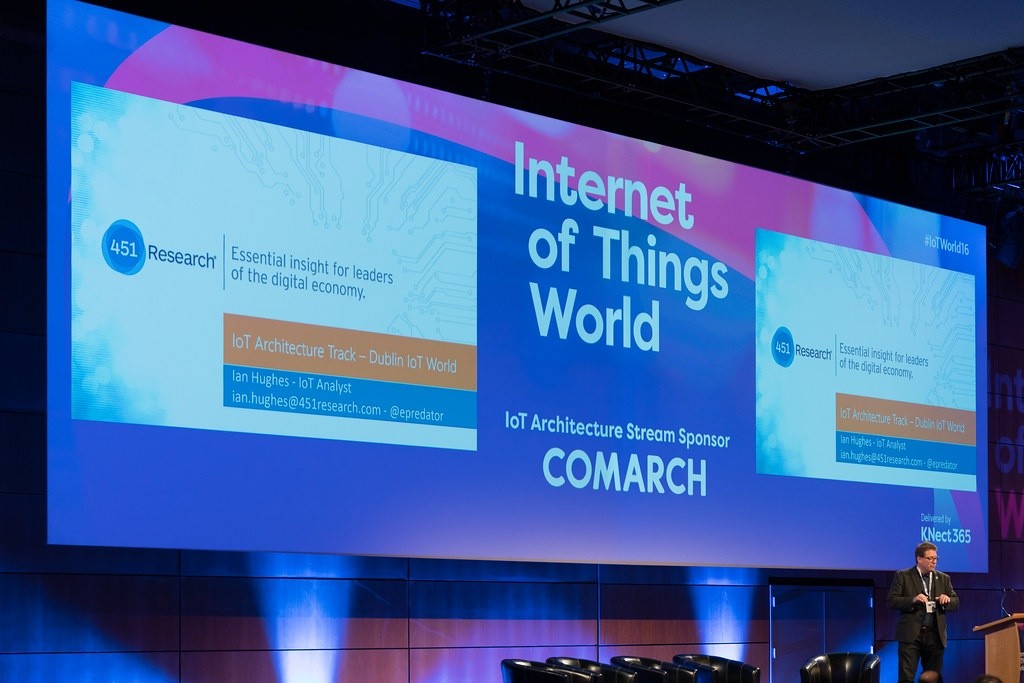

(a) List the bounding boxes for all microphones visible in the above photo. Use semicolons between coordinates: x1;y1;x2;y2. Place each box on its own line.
1000;587;1011;616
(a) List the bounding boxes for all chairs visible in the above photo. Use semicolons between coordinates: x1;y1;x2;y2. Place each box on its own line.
672;653;761;683
501;658;603;683
609;655;698;683
800;652;880;683
546;657;638;683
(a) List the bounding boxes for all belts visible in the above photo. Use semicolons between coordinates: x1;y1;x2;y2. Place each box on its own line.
921;627;937;632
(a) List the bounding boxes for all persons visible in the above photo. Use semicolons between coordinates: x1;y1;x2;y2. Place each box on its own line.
885;542;959;683
918;670;941;683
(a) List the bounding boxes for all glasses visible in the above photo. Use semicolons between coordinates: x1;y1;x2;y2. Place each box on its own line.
919;555;940;561
